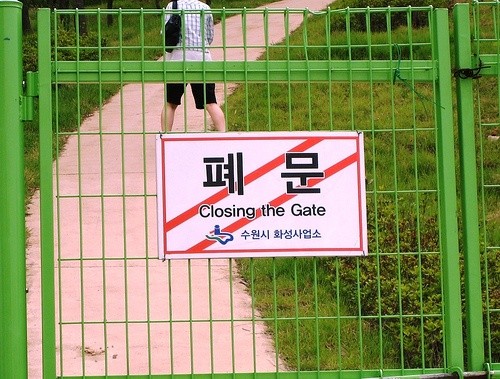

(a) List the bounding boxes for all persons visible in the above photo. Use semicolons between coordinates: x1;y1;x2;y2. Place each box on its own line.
157;0;226;131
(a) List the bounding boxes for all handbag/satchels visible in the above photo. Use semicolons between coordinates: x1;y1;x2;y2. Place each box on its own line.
165;0;181;53
